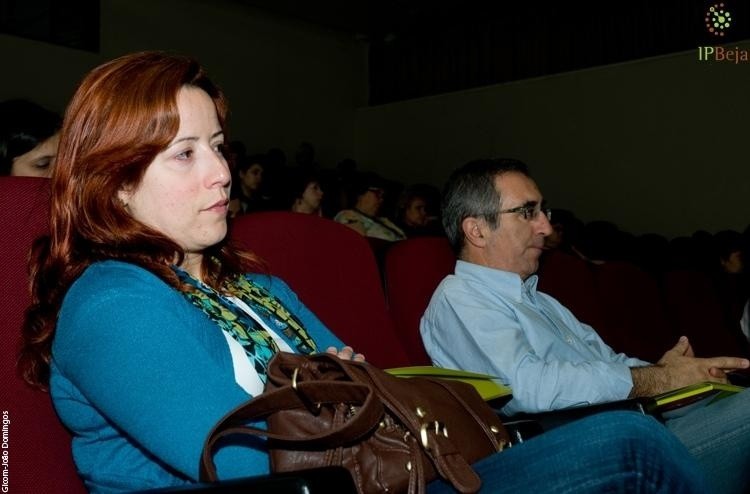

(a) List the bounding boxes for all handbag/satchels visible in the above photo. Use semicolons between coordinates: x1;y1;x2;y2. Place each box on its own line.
196;346;512;494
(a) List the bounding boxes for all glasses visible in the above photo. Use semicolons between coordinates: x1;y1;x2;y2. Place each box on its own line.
368;189;385;199
464;201;554;223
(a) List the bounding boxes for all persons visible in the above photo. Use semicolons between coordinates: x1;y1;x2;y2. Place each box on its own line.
16;52;720;493
418;157;749;493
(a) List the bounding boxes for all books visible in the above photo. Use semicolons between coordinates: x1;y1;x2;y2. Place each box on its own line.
644;380;744;411
386;367;511;405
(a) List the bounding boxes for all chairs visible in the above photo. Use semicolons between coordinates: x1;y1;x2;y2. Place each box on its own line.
2;146;750;493
